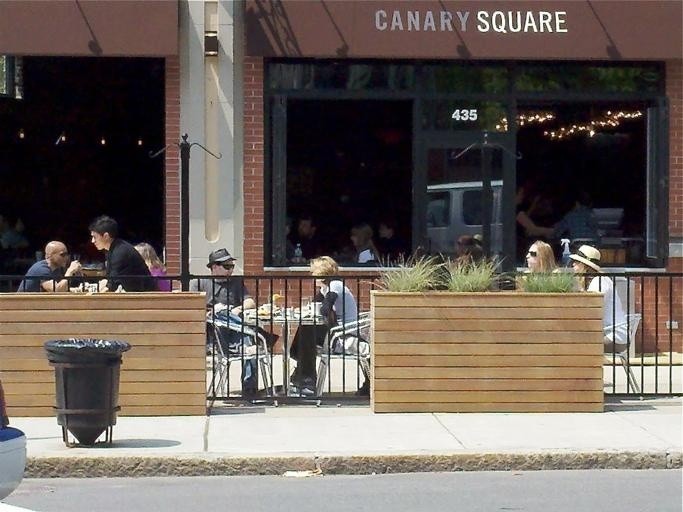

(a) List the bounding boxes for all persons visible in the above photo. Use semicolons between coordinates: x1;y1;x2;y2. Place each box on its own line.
516;186;556;261
188;248;279;406
525;240;559;274
351;225;380;263
473;234;483;247
568;245;631;353
554;196;601;246
289;256;359;389
16;241;82;293
89;216;157;291
134;243;172;292
290;217;317;259
457;236;483;263
379;221;409;262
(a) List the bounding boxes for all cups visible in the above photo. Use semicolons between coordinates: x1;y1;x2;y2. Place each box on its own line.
301;298;310;320
259;304;271;313
88;284;99;295
311;302;322;318
80;283;90;291
282;306;295;317
71;288;80;294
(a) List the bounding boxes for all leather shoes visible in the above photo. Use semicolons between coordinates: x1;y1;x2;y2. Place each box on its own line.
242;385;265;403
255;327;279;348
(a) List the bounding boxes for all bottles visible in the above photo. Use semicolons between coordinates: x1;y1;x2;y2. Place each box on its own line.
295;244;302;263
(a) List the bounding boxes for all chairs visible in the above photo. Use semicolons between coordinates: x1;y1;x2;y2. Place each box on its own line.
315;313;372;405
206;317;280;414
603;313;644;403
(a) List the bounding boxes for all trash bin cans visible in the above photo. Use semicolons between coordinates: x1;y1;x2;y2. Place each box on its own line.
44;335;132;445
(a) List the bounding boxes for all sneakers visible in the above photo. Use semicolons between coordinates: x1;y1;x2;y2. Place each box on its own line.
281;385;299;395
301;385;317;396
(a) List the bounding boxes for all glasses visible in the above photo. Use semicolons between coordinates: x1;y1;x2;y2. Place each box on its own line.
216;263;235;270
53;252;68;257
527;250;537;257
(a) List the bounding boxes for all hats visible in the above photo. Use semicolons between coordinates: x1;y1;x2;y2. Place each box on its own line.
569;245;601;272
206;248;236;268
